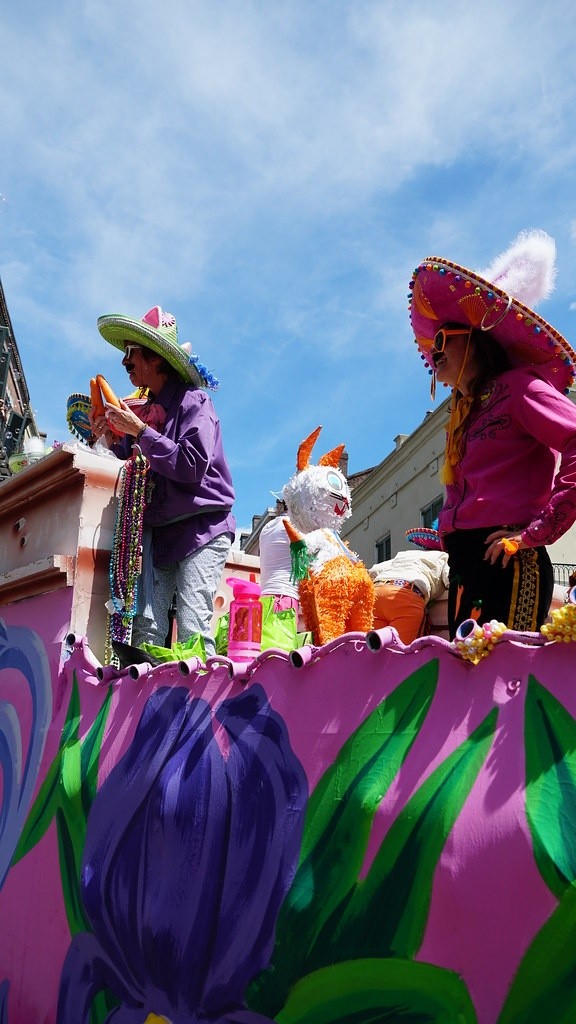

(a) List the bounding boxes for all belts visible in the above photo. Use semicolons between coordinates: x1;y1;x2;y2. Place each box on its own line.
373;579;425;601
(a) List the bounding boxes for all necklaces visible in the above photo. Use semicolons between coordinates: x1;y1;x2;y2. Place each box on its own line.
105;455;147;667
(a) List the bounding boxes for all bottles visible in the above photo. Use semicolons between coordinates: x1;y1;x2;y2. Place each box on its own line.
226;577;264;665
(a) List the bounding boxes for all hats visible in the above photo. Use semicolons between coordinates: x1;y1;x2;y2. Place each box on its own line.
97;306;220;391
407;228;576;398
405;520;443;550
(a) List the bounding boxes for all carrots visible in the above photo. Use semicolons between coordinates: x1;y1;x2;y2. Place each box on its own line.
89;378;105;420
95;374;122;409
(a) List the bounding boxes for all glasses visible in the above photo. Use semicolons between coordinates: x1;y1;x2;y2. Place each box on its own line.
126;345;143;358
433;328;471;352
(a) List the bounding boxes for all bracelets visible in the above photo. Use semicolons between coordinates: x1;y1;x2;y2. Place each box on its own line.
136;423;147;440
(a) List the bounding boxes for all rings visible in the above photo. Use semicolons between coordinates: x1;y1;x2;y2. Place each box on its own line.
94;423;97;427
501;538;519;554
110;419;113;423
99;426;102;429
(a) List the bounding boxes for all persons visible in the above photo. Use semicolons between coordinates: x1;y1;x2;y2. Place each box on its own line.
409;255;575;637
257;516;299;630
88;305;237;668
368;550;450;645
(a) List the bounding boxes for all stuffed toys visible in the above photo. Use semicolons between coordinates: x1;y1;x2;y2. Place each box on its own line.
283;425;376;646
